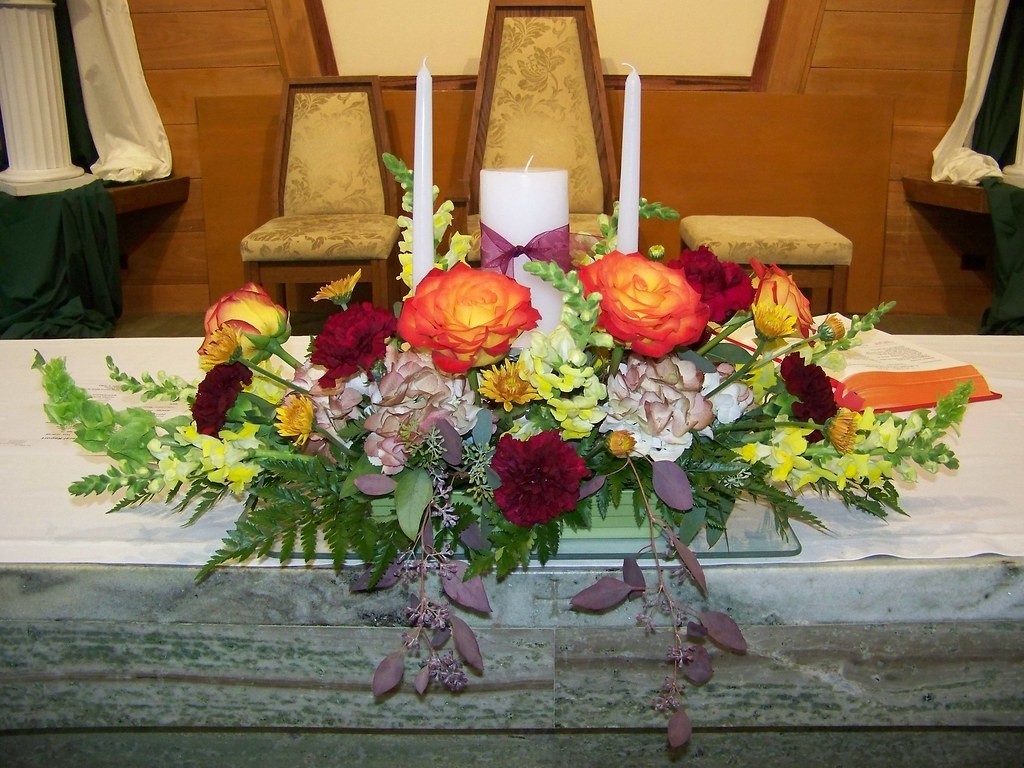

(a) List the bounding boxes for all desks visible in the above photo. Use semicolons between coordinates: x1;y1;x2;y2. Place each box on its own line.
0;331;1024;768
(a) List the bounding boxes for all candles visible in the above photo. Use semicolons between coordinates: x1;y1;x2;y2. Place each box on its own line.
412;56;434;295
617;62;640;255
480;154;570;349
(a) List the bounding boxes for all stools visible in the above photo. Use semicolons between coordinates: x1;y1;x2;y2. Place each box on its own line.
680;215;853;317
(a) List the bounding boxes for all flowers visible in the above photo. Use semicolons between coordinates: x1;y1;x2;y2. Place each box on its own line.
26;149;975;753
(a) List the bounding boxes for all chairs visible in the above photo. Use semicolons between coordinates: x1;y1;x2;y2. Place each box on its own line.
438;0;618;254
240;74;403;314
